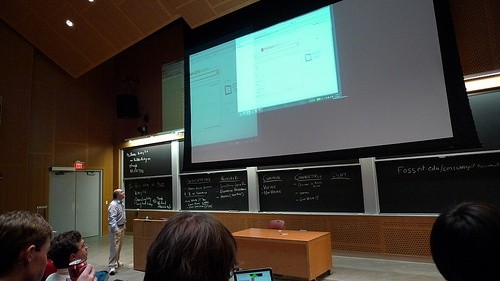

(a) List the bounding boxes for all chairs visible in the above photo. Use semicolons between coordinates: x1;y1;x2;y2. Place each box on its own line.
269;220;285;229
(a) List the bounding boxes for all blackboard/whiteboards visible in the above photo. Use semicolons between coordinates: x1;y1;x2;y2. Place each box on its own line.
179;168;249;212
374;152;500;214
122;140;172;178
124;175;173;211
176;84;500;175
256;164;365;214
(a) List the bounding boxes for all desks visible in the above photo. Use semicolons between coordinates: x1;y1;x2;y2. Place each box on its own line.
231;229;333;281
133;218;170;271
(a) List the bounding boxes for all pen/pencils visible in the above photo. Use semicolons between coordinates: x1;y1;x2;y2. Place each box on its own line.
280;233;288;236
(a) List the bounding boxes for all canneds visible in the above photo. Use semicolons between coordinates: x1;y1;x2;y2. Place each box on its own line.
68;259;87;281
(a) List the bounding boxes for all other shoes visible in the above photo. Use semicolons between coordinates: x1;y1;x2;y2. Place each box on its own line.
109;268;116;275
118;261;124;267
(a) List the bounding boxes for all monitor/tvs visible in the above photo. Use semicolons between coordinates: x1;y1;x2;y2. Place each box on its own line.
234;267;274;281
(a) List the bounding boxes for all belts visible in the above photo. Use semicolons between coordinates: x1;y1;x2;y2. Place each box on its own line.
108;224;125;226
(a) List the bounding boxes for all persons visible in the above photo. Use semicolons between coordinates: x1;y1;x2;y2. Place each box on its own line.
0;210;97;281
47;230;109;281
46;229;98;281
143;212;238;281
428;200;500;281
107;189;126;274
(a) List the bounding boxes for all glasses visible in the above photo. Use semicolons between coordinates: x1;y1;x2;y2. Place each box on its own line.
77;242;87;252
